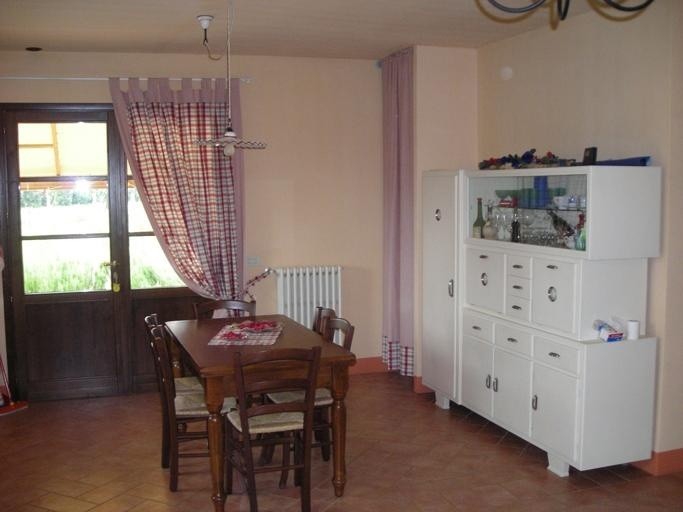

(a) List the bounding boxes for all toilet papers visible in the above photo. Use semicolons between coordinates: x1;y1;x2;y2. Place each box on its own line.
627;320;642;342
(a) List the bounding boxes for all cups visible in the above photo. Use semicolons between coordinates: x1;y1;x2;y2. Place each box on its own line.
519;176;585;209
627;320;640;340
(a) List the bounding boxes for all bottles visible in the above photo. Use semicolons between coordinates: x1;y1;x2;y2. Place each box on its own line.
473;197;585;250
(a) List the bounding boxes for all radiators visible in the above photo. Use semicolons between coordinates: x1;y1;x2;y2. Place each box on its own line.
274;266;343;347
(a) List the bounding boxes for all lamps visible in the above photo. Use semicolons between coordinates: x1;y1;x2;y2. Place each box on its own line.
193;8;267;156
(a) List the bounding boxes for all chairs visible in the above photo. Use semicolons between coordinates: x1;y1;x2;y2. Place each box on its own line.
256;307;336;460
265;315;355;489
225;346;322;512
150;324;238;492
145;315;205;433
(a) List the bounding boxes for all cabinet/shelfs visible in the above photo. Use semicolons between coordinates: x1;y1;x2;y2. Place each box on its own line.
421;173;461;405
